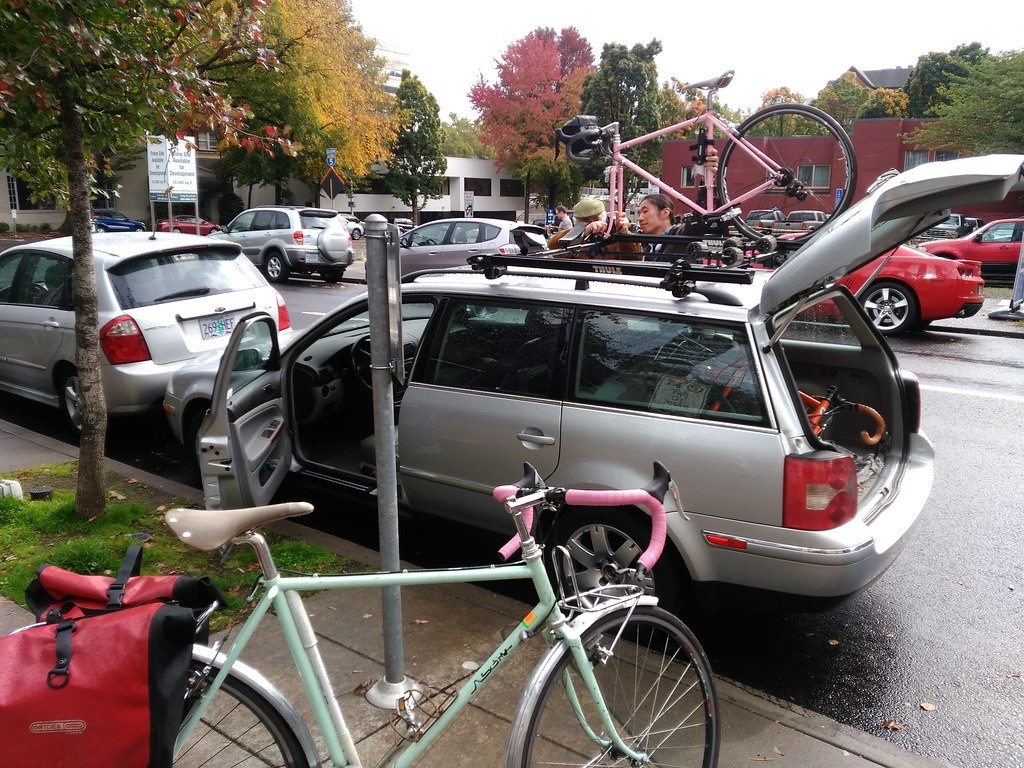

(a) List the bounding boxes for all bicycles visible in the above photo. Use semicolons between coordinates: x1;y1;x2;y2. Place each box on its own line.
654;329;886;444
0;460;720;768
554;70;858;246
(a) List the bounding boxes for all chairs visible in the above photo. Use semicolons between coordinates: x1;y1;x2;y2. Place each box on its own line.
497;310;566;393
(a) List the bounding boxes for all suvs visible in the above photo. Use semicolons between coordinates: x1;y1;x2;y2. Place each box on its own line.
340;214;365;230
205;205;356;283
163;155;1023;643
91;209;147;232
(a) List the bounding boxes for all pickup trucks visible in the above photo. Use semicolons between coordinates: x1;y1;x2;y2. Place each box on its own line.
745;209;832;237
910;214;986;246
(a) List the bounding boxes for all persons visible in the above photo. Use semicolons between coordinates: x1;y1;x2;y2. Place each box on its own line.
555;205;573;233
637;149;720;263
548;199;642;261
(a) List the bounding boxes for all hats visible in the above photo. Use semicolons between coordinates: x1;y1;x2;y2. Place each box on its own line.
573;197;606;219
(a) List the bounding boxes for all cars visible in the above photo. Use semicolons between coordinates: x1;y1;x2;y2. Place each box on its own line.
150;215;221;235
365;217;553;280
394;218;418;235
915;219;1024;266
337;214;364;240
1;232;294;437
697;230;985;334
